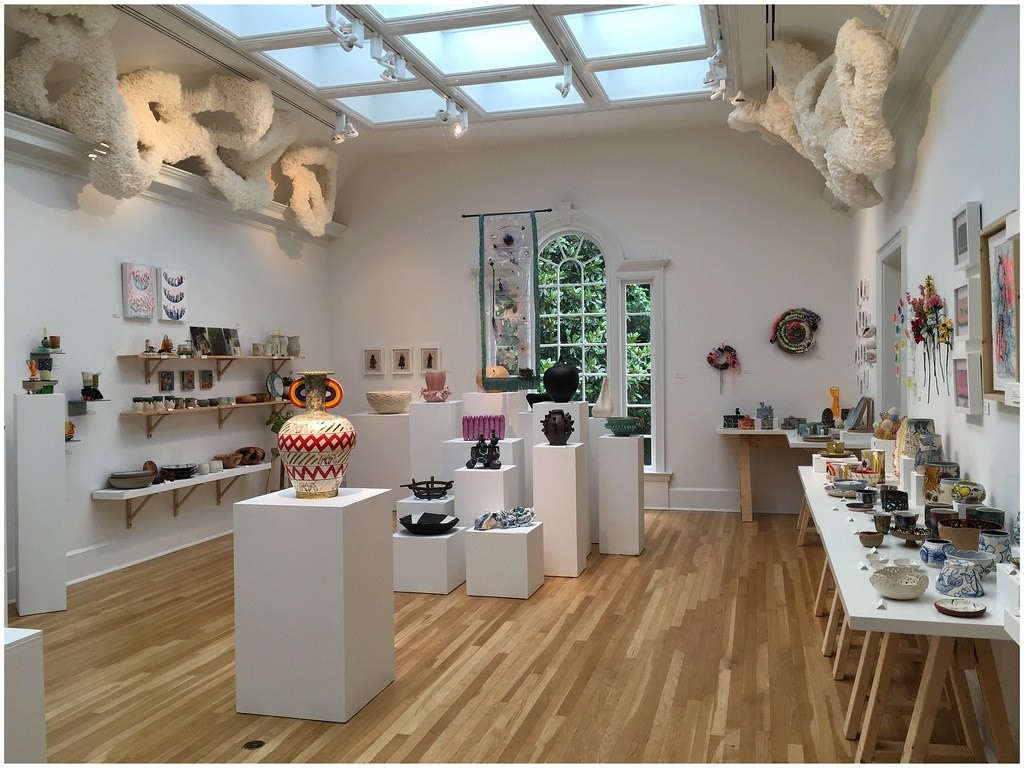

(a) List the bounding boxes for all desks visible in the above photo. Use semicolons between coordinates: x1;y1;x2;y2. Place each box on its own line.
232;392;645;723
799;466;1020;763
716;425;873;524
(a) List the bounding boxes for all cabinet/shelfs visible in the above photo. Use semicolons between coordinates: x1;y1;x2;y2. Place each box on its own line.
457;207;555;393
93;354;304;529
13;392;68;616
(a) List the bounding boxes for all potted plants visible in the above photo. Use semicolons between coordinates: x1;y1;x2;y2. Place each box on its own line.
281;376;293;394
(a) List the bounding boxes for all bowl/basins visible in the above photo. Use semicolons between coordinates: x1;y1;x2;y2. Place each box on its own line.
938;519;1004;549
833;478;869;490
108;470;156;489
860;532;883;548
366;391;459;535
945;549;996;582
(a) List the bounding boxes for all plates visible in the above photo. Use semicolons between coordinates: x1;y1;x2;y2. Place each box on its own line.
162;464;197;479
846;503;875;511
934;598;987;618
825;486;858;497
267;372;283;397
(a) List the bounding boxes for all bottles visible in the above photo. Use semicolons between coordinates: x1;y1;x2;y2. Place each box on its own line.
276;371;357;500
920;538;957;568
934;559;985;598
543;356;614;417
906;419;986;503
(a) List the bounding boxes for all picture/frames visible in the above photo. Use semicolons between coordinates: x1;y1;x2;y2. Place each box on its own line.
364;349;384;375
392;348;412;375
158;370;213;391
419;347;440;373
849;396;867;429
948;200;1020;414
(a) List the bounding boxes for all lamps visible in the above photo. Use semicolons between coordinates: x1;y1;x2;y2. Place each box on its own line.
556;65;572;98
312;5;409;83
88;142;111;158
330;98;468;146
703;42;748;106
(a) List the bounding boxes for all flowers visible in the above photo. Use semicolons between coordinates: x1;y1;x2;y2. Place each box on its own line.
892;275;954;405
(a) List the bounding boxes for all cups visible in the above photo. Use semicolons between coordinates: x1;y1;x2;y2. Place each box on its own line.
978;530;1011;571
925;502;953;528
798;423;828;436
826;460;847;483
132;342;275;475
975;507;1005;525
895;511;919;528
874;514;890;535
930;508;959;530
855;490;877;505
861;450;885;483
850;470;878;487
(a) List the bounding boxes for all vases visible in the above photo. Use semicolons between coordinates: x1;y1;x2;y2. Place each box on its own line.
274;370;357;499
